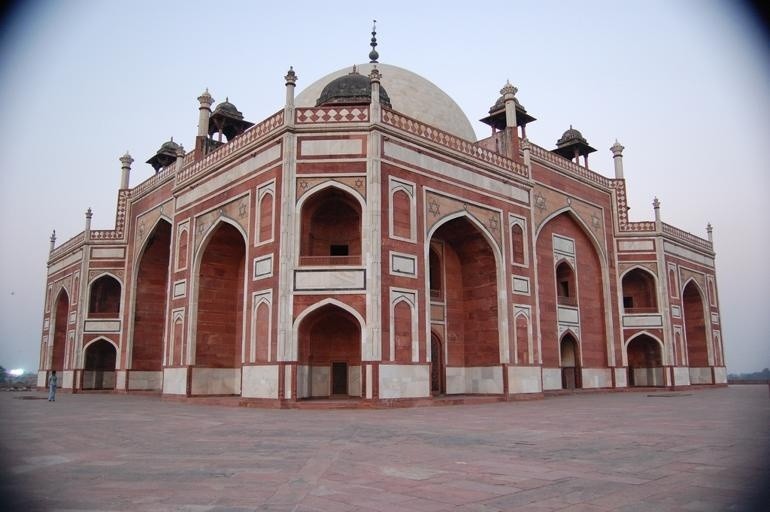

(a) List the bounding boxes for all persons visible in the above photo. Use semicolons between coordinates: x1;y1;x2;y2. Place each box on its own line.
48;371;57;401
